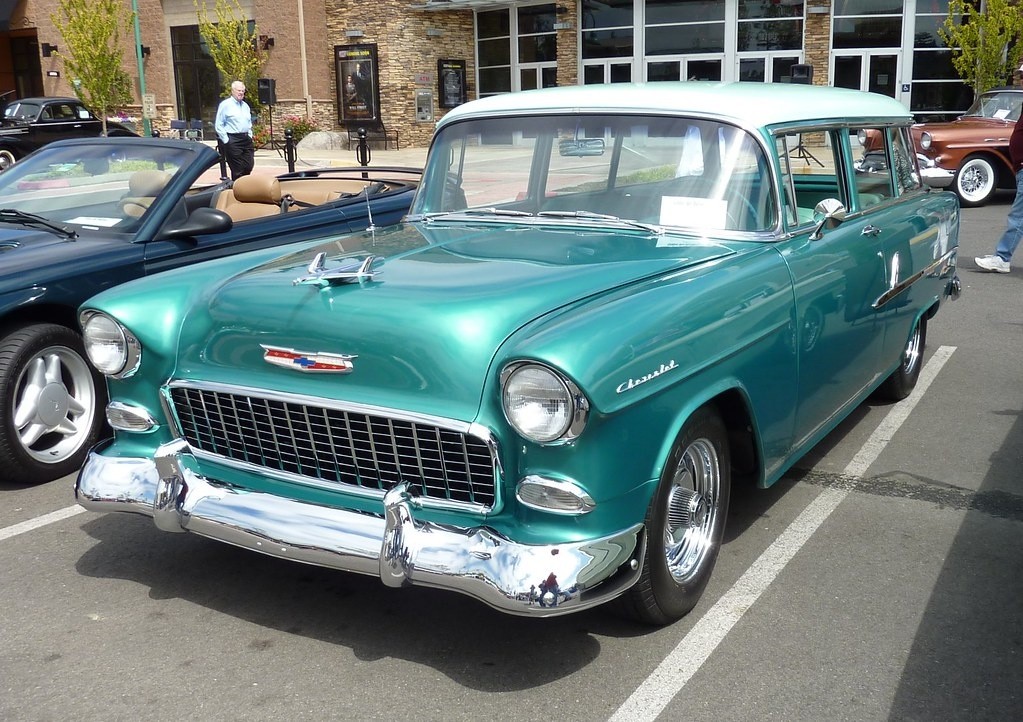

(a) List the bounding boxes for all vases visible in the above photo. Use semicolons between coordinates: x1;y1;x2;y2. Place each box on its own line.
117;122;136;133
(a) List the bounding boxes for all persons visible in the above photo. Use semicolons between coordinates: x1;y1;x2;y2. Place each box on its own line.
975;63;1022;272
215;81;256;181
345;63;372;105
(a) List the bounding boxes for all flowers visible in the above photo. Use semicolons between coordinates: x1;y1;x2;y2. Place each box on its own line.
105;110;138;124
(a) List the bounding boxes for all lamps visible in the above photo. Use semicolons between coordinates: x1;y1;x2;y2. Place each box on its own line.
808;6;829;13
135;44;151;59
554;23;571;30
258;34;275;52
42;42;58;58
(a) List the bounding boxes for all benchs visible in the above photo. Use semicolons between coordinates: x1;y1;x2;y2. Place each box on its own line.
345;120;400;151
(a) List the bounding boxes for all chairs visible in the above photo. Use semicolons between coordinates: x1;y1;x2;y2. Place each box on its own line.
224;172;283;221
120;166;175;218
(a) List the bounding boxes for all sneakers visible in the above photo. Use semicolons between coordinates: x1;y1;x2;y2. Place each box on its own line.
974;255;1011;273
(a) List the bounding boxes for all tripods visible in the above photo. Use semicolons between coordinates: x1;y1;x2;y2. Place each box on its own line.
779;132;824;168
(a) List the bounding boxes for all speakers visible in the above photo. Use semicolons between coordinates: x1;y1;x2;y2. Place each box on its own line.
790;64;813;85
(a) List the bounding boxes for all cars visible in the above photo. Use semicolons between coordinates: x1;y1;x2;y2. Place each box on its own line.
0;97;142;172
0;137;466;483
74;82;962;625
854;84;1023;207
